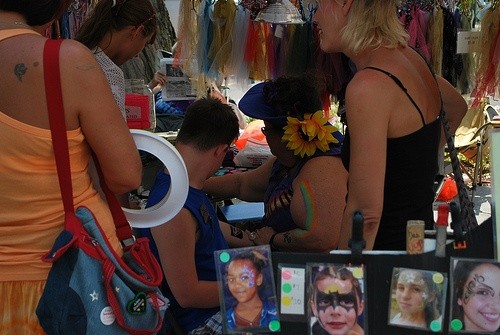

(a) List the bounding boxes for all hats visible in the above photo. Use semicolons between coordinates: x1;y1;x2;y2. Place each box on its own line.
238;82;290;119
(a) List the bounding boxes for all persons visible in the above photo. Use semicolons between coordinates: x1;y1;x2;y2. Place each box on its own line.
452;260;500;333
73;0;159;200
0;0;143;335
202;74;350;250
390;269;441;328
311;267;365;335
312;0;468;251
225;251;278;331
138;95;260;335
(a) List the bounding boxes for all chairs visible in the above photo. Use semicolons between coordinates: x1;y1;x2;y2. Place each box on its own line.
434;98;487;211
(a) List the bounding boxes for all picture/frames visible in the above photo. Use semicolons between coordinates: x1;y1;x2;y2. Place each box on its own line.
212;243;500;335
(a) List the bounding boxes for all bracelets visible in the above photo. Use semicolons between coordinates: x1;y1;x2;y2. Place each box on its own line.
269;232;279;249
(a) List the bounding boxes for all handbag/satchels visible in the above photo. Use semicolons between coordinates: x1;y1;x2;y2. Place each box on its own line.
35;205;169;335
